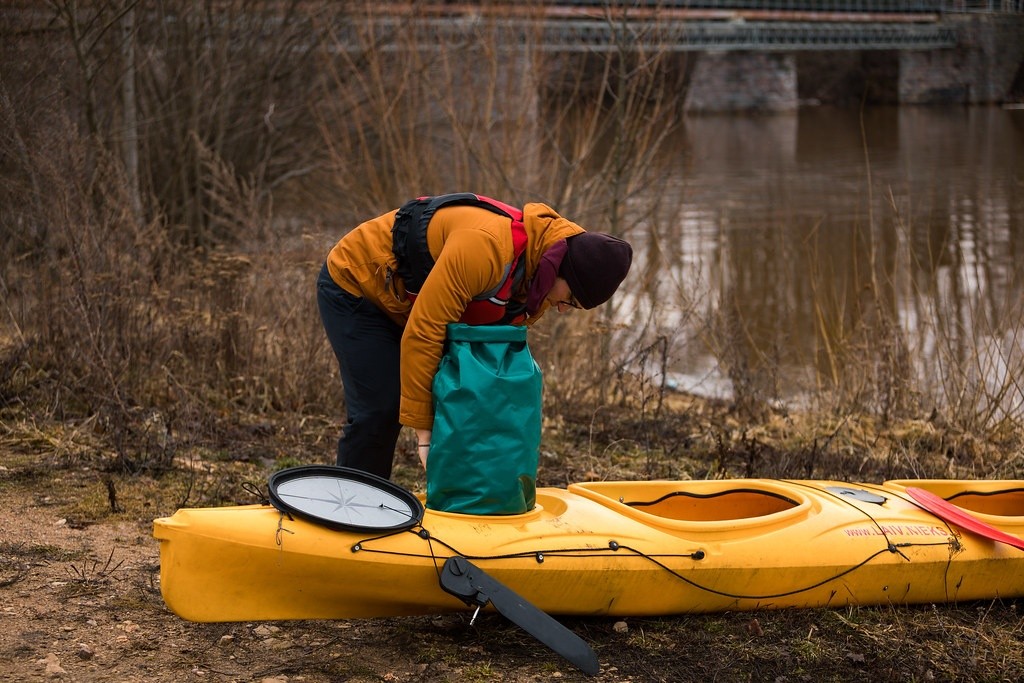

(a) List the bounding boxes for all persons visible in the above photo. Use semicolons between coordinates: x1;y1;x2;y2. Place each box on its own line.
316;192;635;482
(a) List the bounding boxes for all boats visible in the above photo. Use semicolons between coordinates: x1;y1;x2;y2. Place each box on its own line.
153;479;1024;623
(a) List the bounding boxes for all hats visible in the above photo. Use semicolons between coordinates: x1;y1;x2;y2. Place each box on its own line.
560;232;633;310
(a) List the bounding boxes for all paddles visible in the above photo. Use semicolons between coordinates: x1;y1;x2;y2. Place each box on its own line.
905;486;1024;552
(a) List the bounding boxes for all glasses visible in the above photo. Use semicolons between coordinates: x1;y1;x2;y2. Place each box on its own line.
561;292;582;309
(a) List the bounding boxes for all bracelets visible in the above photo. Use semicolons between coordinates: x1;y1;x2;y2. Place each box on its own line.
418;444;431;448
